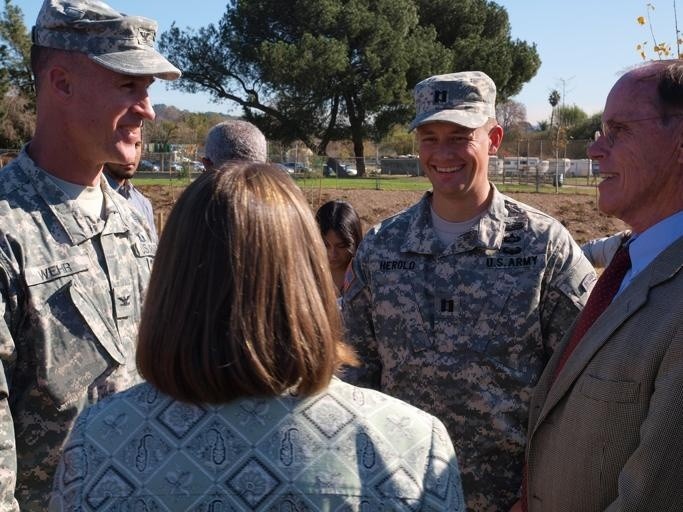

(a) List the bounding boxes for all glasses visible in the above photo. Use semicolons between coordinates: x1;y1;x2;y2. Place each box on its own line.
593;108;677;147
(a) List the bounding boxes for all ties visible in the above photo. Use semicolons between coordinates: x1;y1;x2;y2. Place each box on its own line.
522;234;631;510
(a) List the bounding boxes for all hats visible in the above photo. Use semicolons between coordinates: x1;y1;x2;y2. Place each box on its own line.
31;0;182;83
406;70;496;133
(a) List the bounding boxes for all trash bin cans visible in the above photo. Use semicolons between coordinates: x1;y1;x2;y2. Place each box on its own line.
553;174;564;187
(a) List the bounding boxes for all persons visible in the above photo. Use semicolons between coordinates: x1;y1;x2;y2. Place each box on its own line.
0;0;186;512
314;200;364;306
512;58;683;512
200;119;270;169
49;155;468;512
331;68;605;511
576;229;636;271
98;118;157;223
